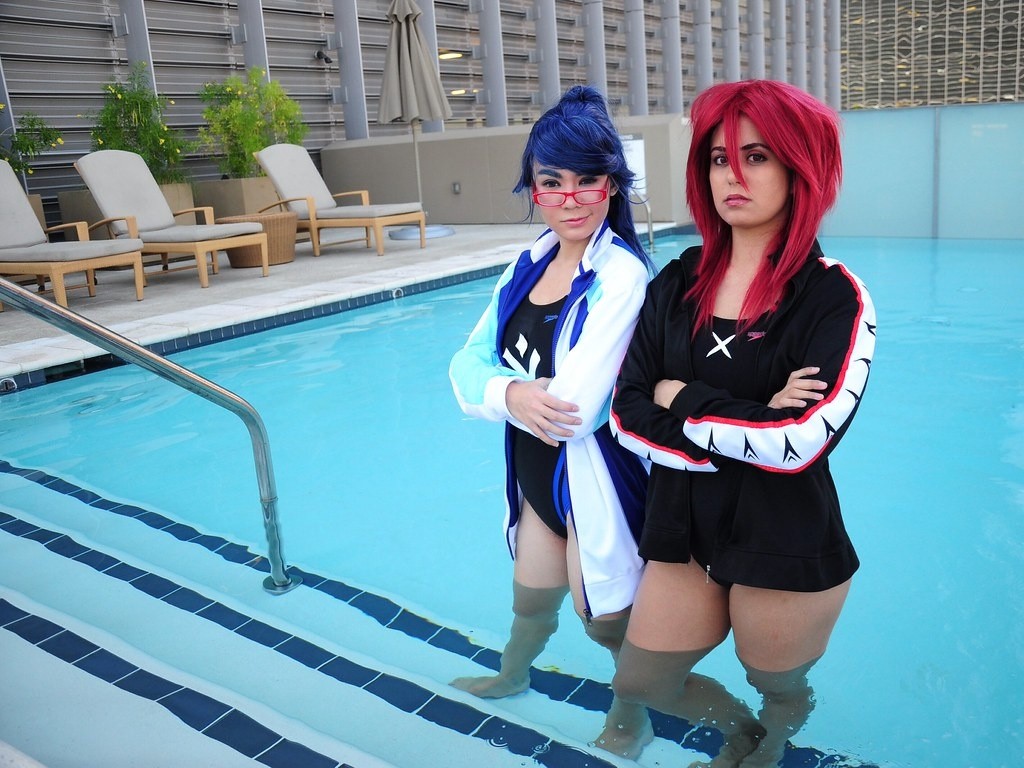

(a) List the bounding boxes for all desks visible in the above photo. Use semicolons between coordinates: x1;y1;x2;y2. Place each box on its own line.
216;212;297;267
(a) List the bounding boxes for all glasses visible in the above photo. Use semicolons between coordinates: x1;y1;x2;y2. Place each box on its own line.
532;176;611;209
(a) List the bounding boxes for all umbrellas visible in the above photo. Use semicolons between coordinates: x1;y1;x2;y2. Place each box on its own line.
377;0;453;209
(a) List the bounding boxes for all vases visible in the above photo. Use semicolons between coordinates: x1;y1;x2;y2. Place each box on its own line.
195;178;287;223
57;183;197;270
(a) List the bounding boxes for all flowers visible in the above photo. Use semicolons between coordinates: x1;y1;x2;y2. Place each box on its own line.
191;66;313;180
0;103;64;174
57;62;191;184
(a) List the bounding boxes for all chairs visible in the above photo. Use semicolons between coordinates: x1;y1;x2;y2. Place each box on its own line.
73;149;270;288
0;158;144;313
254;143;427;257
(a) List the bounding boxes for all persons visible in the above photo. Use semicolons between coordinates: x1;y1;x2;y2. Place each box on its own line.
609;79;875;768
448;84;655;762
